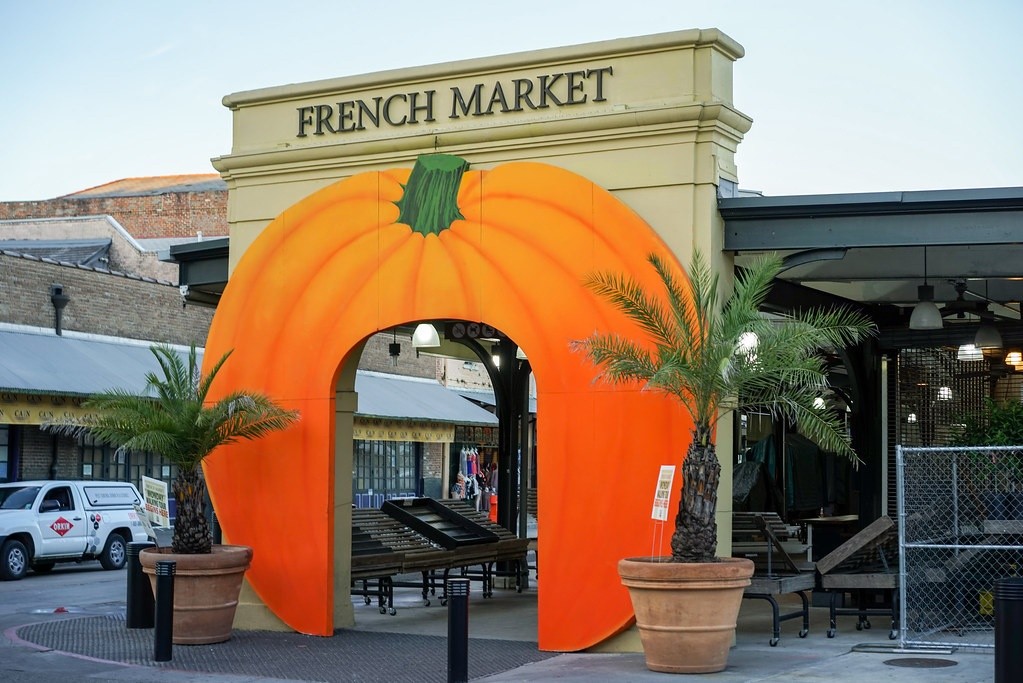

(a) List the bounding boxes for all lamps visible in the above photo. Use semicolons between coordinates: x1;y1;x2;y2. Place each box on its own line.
938;386;953;400
412;324;442;347
957;344;983;361
974;279;1003;349
908;246;944;330
1004;348;1023;371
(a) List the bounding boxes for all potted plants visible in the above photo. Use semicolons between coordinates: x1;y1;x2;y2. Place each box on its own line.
37;340;305;645
565;241;879;674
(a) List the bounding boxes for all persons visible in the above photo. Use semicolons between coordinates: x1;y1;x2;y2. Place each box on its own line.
459;446;498;512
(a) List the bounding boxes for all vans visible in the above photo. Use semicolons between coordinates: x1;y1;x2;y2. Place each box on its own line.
0;481;149;581
